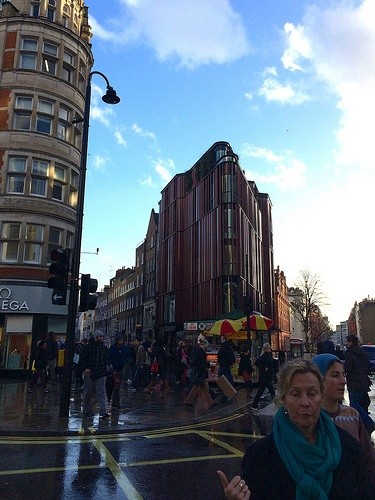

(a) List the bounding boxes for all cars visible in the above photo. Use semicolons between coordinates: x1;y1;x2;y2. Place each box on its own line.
360;345;375;372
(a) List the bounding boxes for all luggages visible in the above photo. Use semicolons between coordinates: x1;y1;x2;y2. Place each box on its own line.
208;370;237;401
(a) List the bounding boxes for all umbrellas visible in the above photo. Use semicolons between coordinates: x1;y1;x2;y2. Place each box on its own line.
209;315;243;343
235;314;274;335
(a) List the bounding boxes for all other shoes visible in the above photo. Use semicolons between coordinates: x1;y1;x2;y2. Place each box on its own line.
27;387;33;394
99;413;108;418
83;412;94;419
209;403;218;409
367;424;375;433
252;404;261;411
44;388;49;393
184;403;193;407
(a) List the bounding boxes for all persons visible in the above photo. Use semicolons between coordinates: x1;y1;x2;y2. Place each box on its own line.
27;326;251;417
305;354;375;465
332;340;370;360
217;357;374;500
252;342;278;412
341;334;373;416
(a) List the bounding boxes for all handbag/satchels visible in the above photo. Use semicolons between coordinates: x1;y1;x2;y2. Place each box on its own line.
73;344;81;364
150;357;158;372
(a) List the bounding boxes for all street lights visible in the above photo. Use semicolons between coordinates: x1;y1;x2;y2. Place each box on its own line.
58;70;122;419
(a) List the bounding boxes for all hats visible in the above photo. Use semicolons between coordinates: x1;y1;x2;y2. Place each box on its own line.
94;330;104;338
311;353;342;378
115;334;123;341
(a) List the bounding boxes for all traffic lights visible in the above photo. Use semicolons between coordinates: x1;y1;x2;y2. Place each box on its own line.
47;249;69;306
78;274;98;312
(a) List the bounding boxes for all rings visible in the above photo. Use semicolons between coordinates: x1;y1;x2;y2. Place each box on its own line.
241;488;247;494
238;483;245;489
